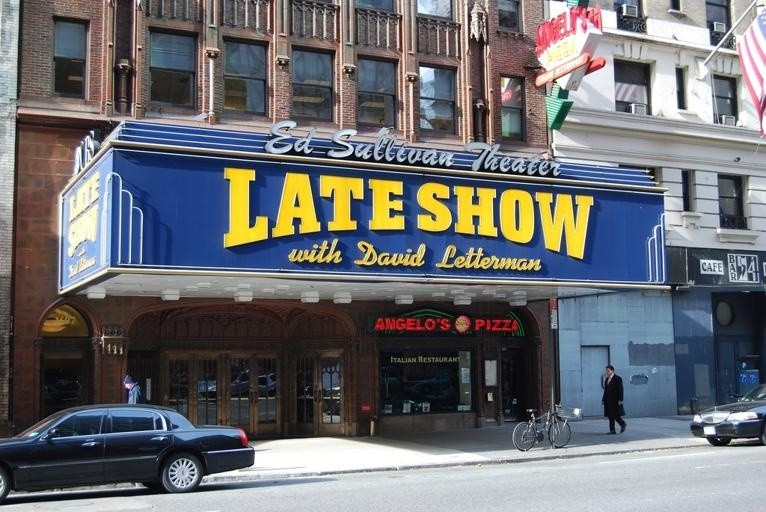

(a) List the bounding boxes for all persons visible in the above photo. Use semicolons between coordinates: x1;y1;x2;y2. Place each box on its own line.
600;364;628;434
122;373;146;405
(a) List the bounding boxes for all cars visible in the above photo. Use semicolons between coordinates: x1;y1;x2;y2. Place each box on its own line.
1;404;256;504
170;369;276;397
379;365;454;396
694;383;766;445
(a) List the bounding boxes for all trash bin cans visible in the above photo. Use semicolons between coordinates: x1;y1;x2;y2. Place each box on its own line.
368;415;379;437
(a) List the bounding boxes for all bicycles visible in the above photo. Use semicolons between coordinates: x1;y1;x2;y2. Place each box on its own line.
511;400;573;450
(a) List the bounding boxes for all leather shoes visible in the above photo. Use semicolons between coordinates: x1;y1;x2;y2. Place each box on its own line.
621;423;626;432
607;431;616;434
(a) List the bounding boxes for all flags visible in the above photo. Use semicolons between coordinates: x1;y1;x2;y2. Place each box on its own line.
734;6;766;140
